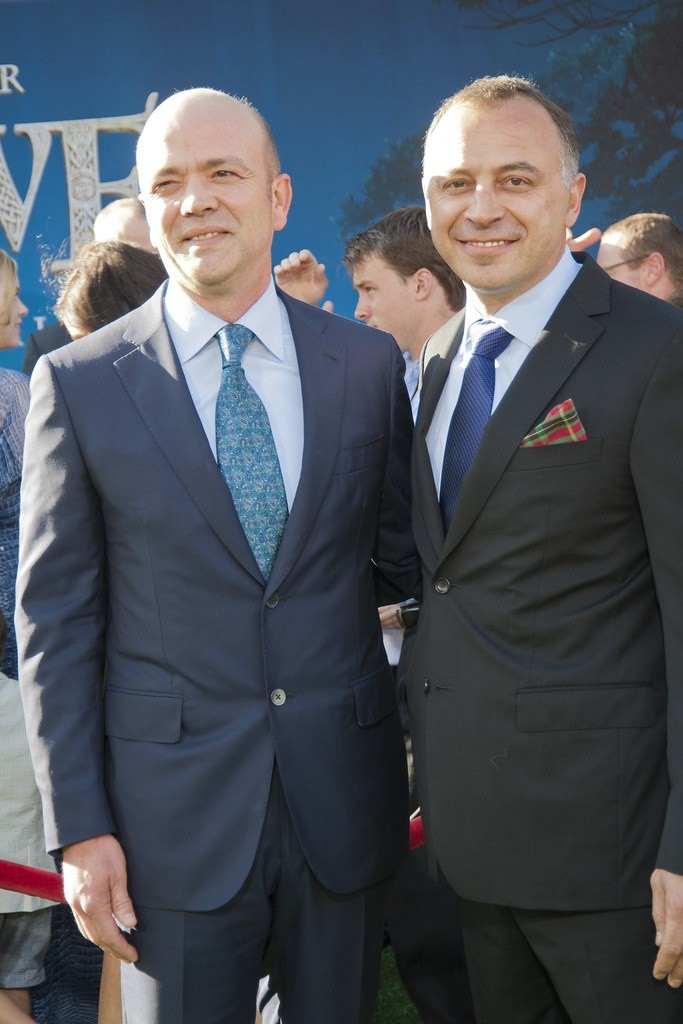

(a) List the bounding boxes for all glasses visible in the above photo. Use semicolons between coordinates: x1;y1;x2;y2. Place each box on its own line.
603;253;651;271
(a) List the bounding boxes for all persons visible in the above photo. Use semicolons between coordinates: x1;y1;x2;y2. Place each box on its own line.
400;78;683;1023
272;209;467;1024
566;213;682;309
0;198;167;1024
12;87;410;1024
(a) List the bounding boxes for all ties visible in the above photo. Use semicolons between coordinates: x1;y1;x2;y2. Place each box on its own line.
438;317;516;538
214;323;290;580
